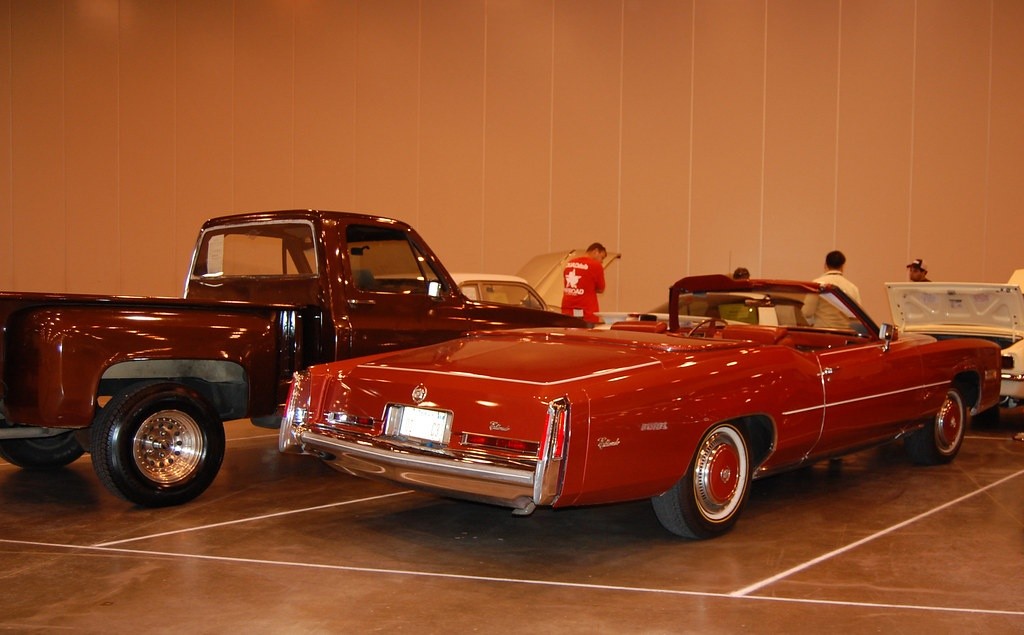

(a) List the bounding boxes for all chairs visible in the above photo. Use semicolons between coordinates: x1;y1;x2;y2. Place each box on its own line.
610;321;669;333
714;325;796;349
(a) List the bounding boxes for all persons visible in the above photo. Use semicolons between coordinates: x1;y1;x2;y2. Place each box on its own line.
800;251;861;330
560;242;608;323
906;258;933;282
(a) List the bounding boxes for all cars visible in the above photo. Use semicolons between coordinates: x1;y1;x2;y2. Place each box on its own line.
278;275;1003;540
594;289;810;337
445;248;622;332
884;267;1024;425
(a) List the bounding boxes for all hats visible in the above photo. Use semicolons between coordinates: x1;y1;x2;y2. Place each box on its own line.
907;259;928;272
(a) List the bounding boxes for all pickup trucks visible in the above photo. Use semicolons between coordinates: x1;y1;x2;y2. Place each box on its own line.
0;209;584;510
